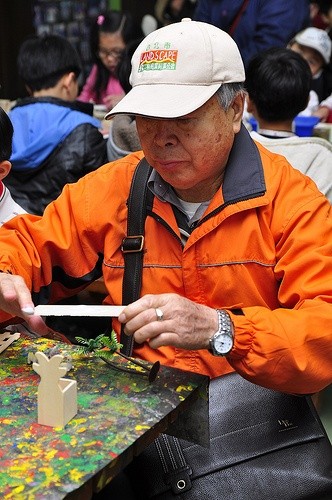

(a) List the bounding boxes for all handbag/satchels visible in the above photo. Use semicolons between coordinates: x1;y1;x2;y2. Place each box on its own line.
125;368;331;500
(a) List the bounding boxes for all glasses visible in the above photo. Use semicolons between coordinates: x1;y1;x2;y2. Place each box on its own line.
99;46;124;60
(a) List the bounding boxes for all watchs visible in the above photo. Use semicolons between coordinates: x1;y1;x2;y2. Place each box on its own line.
207;308;234;357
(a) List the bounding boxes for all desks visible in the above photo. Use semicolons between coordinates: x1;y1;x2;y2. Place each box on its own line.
0;326;206;500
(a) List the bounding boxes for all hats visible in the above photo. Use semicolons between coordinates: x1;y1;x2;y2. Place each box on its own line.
296;29;331;65
106;18;245;121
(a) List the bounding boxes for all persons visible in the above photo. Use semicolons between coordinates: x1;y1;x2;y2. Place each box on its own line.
192;0;310;64
3;36;109;216
285;27;331;79
0;17;332;412
0;106;29;227
74;10;146;113
244;47;332;204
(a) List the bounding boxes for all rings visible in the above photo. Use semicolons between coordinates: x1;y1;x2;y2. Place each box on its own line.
154;308;164;322
